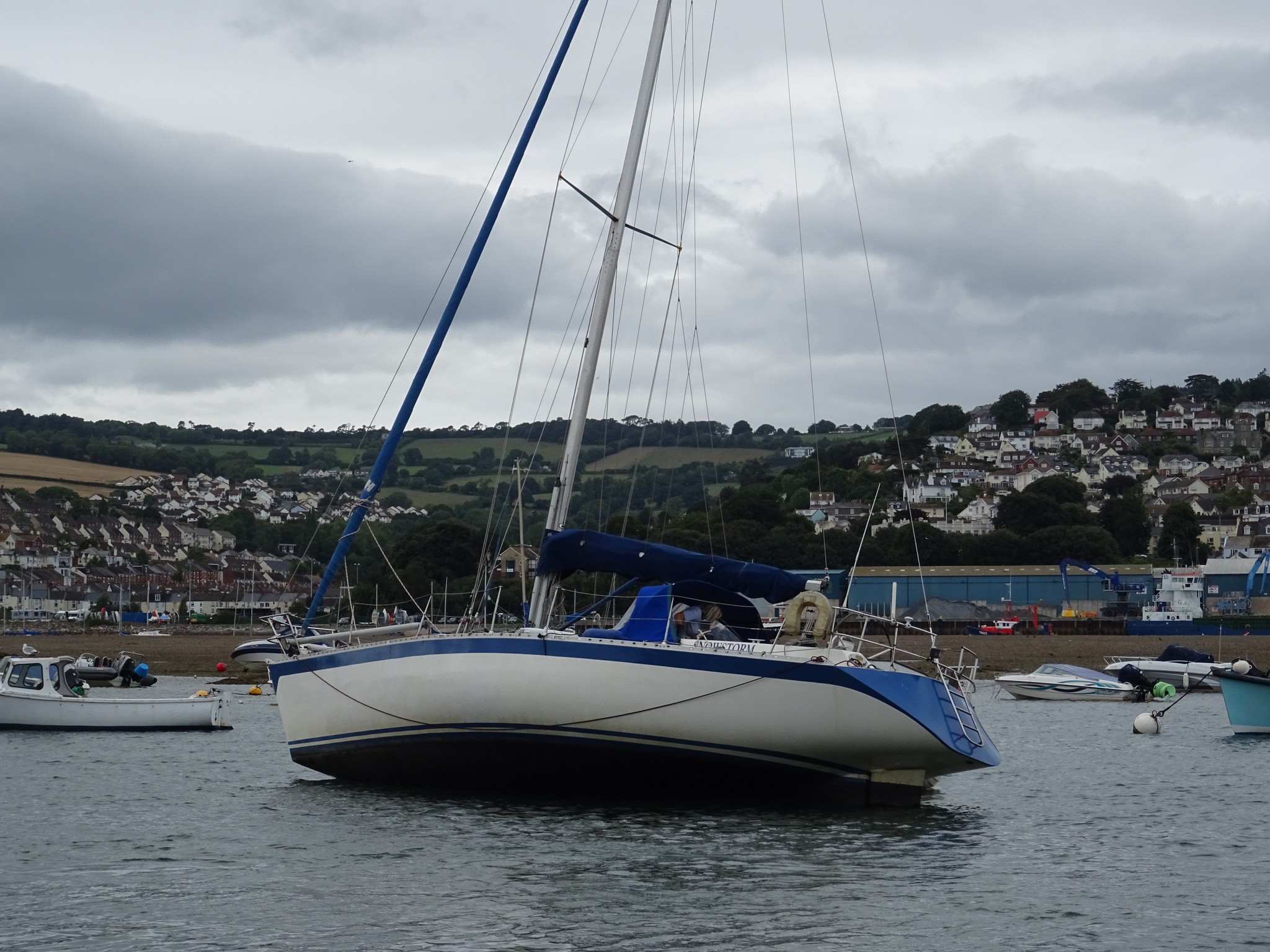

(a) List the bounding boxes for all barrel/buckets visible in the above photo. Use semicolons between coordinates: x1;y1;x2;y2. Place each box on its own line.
135;663;148;677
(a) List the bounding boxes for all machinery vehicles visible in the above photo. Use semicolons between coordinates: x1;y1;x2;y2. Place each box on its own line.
1217;548;1270;615
1059;558;1146;617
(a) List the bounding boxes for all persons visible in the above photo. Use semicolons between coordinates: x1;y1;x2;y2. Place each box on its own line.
701;606;743;642
673;595;711;645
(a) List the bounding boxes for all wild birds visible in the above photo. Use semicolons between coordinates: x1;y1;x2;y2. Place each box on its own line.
23;643;38;657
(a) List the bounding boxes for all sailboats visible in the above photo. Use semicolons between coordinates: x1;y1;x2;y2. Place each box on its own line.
265;1;1002;795
980;567;1020;635
118;581;171;636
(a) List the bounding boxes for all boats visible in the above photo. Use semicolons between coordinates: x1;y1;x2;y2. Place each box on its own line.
980;568;1020;635
967;625;997;635
8;609;53;622
0;653;234;729
1210;657;1270;735
994;662;1138;702
231;638;352;668
52;610;90;622
73;654;129;688
1102;644;1232;693
147;610;174;624
